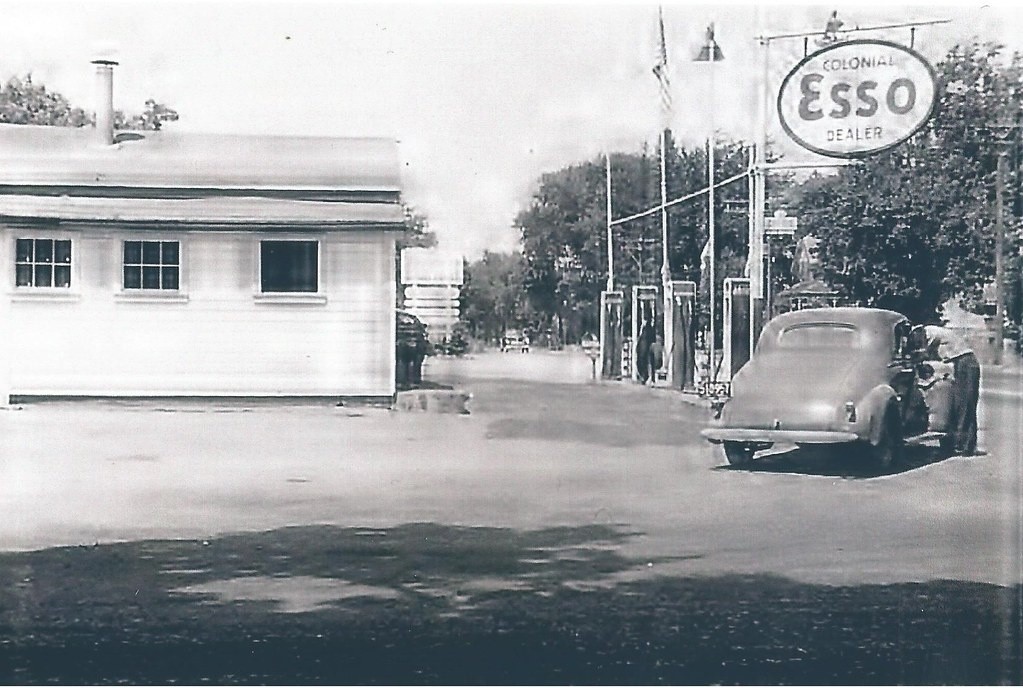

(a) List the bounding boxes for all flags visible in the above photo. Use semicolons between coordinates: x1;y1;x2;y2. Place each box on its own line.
648;7;673;113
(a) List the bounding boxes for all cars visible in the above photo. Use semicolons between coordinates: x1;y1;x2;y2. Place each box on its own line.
499;330;530;353
700;306;928;475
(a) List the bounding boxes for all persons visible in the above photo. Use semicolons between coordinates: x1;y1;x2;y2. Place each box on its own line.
900;325;980;457
637;316;658;387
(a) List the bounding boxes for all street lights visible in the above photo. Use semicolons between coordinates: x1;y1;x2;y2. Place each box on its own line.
992;139;1016;365
694;23;726;384
